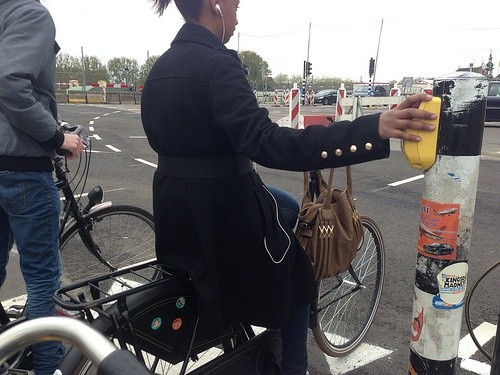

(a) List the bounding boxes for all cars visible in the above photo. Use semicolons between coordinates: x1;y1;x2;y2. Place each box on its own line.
485;80;500;128
307;88;338;105
349;84;388;97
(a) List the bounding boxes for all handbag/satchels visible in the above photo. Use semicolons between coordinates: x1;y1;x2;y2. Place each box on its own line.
296;165;364;278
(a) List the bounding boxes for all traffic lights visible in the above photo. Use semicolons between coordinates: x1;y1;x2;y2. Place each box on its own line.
305;61;312;75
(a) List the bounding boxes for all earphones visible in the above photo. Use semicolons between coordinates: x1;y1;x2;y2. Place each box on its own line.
216;4;223;15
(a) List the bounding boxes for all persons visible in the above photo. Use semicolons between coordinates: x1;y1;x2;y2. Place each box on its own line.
140;0;437;375
0;0;85;375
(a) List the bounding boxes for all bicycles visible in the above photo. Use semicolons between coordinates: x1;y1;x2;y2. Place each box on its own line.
0;116;388;375
